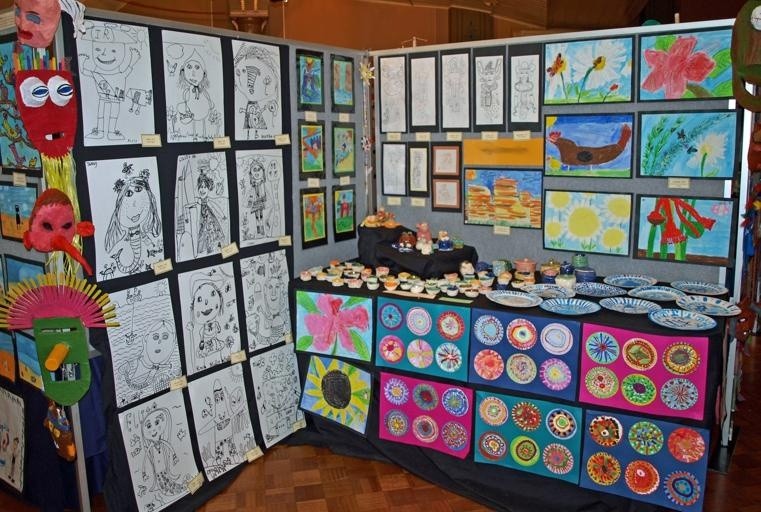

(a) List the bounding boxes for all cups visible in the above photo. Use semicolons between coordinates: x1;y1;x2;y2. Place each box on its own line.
299;260;534;299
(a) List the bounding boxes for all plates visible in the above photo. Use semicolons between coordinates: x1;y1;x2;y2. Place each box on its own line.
485;272;743;332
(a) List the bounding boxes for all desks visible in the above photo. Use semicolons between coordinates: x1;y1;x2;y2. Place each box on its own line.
289;249;730;512
